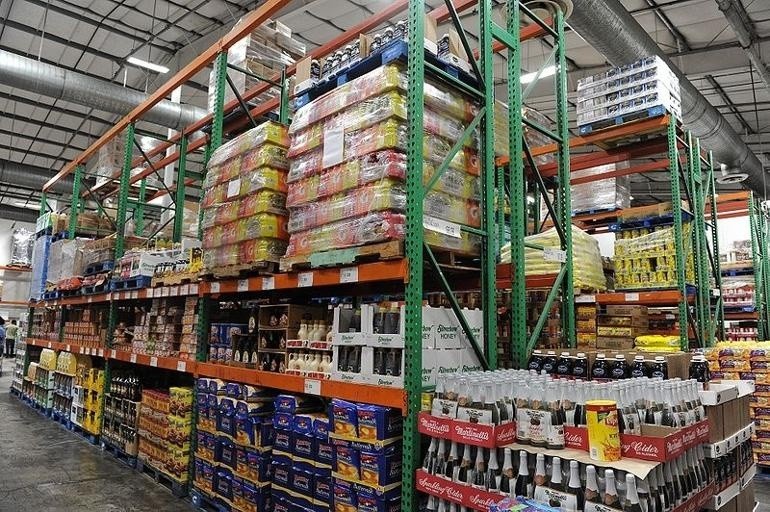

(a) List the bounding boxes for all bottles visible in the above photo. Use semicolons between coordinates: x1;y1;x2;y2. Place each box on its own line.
288;293;401;377
26;350;142;457
419;347;756;512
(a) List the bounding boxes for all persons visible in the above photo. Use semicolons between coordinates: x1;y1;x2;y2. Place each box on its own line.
4;319;18;358
0;316;8;377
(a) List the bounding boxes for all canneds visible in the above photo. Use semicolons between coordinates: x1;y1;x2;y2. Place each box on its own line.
585;400;622;462
